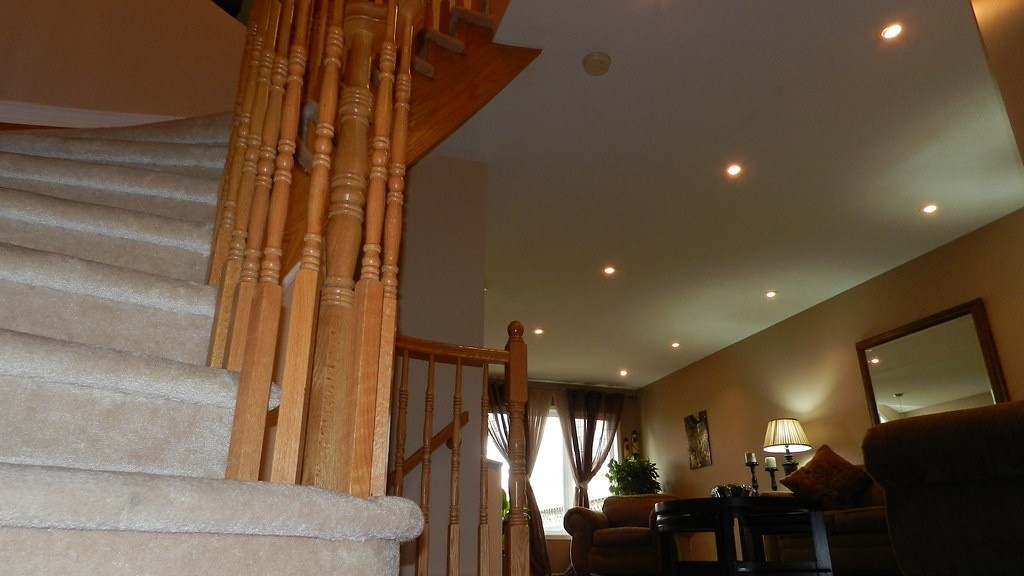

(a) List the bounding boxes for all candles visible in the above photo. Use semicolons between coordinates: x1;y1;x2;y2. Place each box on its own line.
765;456;777;468
744;451;757;462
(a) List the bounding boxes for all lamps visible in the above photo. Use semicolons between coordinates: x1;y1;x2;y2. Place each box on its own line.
764;418;811;491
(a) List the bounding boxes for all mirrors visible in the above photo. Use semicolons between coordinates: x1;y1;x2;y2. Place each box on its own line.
854;300;1009;432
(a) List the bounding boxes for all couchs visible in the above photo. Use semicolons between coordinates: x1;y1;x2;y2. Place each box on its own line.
563;494;691;574
762;398;1024;576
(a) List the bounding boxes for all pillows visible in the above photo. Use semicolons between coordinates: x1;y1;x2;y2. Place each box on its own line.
778;443;871;510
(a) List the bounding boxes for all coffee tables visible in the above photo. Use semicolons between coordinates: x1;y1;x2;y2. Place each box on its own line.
652;494;829;575
